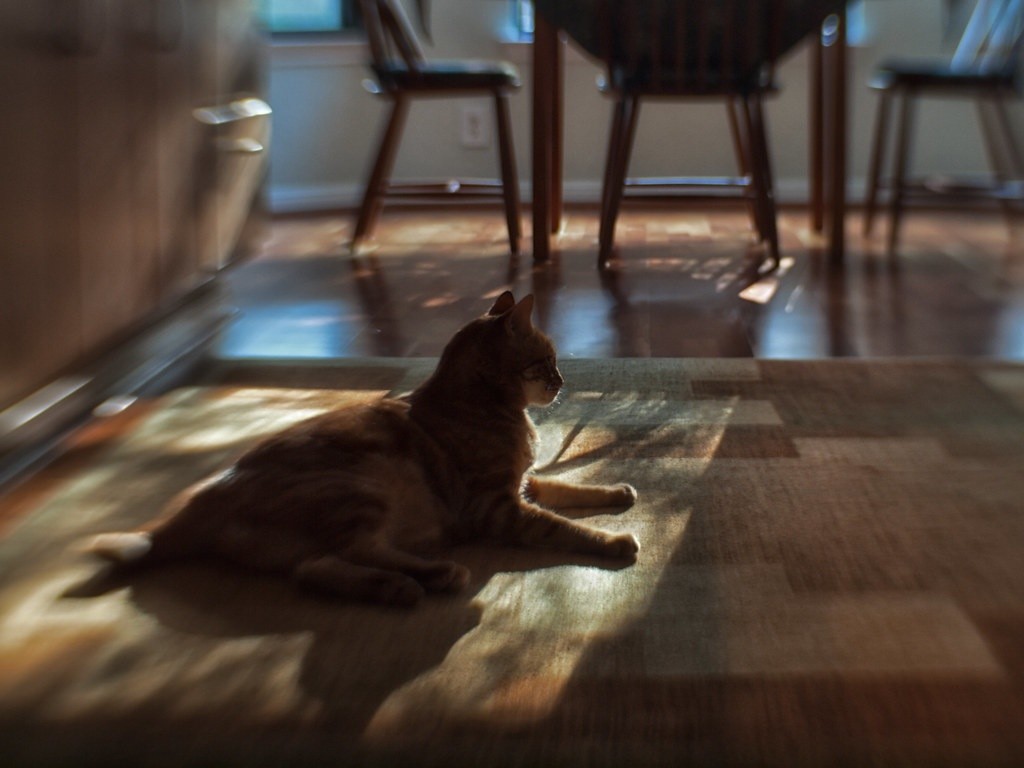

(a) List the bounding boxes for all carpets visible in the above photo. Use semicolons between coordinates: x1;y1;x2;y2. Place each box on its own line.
0;353;1024;766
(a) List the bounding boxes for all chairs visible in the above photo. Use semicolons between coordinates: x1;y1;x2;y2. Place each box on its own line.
598;0;779;270
346;0;522;261
863;0;1023;247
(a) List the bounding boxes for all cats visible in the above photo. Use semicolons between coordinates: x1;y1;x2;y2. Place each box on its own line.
74;288;642;608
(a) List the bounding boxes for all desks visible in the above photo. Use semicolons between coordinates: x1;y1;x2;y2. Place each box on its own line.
527;0;853;269
